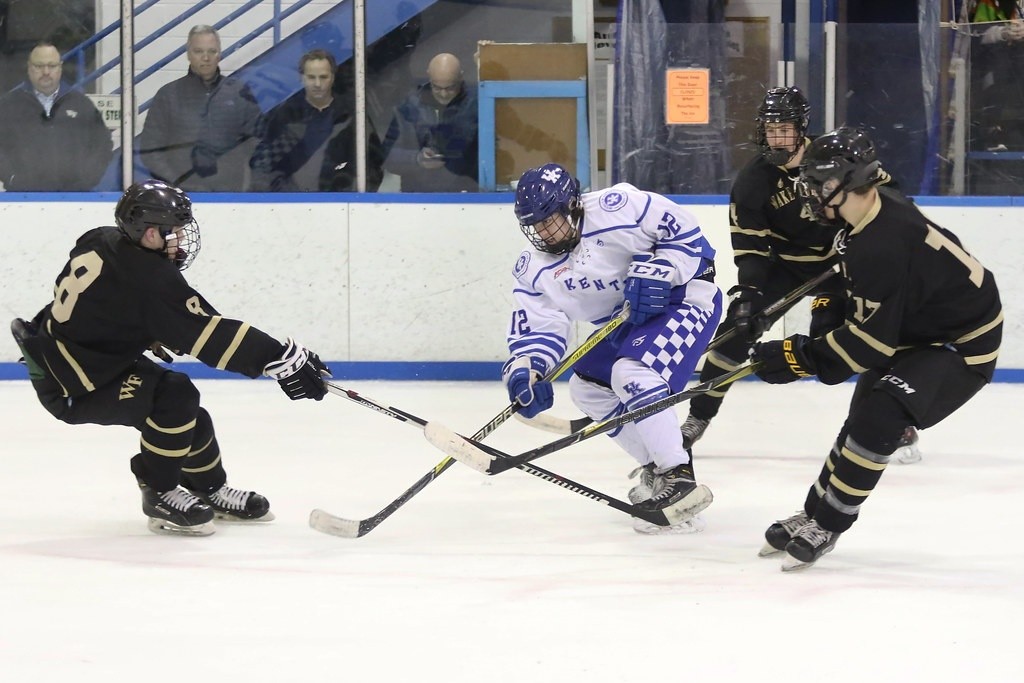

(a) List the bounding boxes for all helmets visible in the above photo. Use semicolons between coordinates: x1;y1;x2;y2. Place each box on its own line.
514;164;583;255
755;87;811;166
114;179;201;272
795;127;882;229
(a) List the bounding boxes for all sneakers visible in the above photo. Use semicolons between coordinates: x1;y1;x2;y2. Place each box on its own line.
985;130;1009;151
890;425;923;466
781;516;841;571
757;509;812;558
631;448;706;535
628;461;658;491
188;483;275;525
680;413;711;451
130;453;217;536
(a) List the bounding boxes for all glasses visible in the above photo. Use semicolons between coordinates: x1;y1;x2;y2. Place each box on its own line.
30;62;61;72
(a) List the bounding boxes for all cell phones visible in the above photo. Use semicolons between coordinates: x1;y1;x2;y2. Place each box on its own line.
431;154;444;159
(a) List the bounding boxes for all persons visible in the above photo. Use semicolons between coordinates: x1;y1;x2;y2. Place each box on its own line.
0;45;114;191
252;49;383;193
11;179;333;535
481;61;568;185
682;88;922;464
971;0;1024;102
503;165;721;533
383;53;477;192
749;129;1003;570
140;25;264;192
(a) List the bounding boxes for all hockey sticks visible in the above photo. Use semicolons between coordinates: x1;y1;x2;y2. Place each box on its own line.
707;261;844;353
421;360;767;478
320;380;712;536
310;304;630;539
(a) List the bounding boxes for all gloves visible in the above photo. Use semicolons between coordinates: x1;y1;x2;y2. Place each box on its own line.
501;353;554;419
809;292;851;338
623;252;677;326
749;333;818;385
727;284;772;345
262;336;333;402
